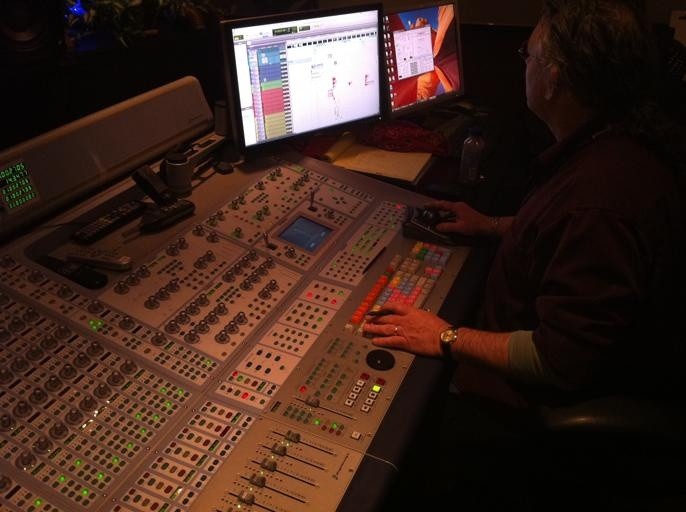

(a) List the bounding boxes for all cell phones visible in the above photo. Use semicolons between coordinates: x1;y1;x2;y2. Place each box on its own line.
69;265;107;289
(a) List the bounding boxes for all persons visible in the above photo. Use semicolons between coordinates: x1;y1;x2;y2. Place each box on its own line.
361;0;685;512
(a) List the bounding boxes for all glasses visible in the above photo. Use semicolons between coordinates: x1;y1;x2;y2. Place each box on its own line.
518;48;549;65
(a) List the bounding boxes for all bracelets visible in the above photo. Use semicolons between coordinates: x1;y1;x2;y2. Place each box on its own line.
486;213;498;234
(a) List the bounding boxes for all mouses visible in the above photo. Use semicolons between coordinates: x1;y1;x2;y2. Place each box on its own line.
403;206;466;245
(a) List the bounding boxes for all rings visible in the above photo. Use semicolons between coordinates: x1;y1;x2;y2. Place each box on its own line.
393;325;400;336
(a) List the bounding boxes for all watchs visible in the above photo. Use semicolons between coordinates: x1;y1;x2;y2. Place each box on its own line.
440;324;462;358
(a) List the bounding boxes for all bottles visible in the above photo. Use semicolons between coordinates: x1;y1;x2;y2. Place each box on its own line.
458;126;485;187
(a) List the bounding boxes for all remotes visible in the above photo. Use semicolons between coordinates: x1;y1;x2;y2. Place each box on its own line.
66;247;131;271
71;199;146;246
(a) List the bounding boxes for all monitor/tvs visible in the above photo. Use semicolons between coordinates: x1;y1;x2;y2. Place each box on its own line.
381;0;466;120
219;2;384;156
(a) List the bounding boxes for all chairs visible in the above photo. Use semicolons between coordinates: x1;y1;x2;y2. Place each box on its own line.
536;392;686;512
